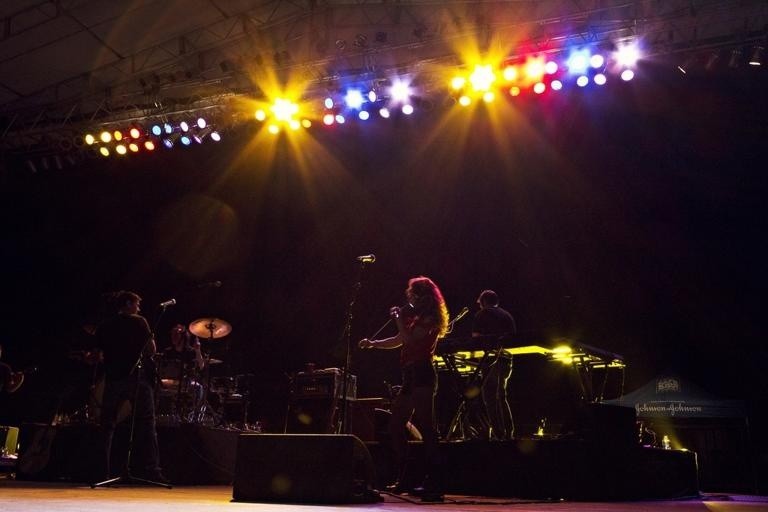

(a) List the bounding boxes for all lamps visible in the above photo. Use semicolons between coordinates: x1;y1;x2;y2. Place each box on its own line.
84;117;226;157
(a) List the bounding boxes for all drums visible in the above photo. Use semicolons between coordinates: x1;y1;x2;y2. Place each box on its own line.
154;379;203;423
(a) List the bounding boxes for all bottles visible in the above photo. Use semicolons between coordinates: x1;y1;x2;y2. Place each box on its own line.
662;436;671;450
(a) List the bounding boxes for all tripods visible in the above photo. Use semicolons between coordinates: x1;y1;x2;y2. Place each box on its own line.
92;307;175;489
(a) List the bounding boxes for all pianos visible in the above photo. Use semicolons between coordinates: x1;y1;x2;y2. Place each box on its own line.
549;337;626;368
433;335;554;374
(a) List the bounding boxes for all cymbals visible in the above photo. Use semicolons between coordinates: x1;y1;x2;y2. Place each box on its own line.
193;358;223;365
189;317;232;338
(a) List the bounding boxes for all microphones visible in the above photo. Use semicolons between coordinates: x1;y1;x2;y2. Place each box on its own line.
198;280;221;289
399;303;414;315
159;299;176;307
456;307;469;321
358;254;376;263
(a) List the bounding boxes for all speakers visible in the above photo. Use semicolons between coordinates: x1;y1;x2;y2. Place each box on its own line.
15;422;130;483
563;400;636;449
234;432;377;502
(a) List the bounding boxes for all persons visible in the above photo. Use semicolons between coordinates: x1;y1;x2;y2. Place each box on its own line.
82;289;172;484
471;288;518;440
157;318;205;380
0;341;25;394
356;274;449;495
